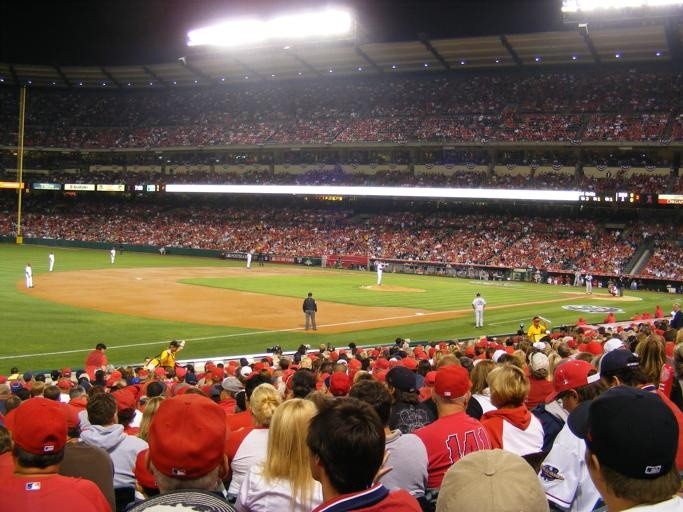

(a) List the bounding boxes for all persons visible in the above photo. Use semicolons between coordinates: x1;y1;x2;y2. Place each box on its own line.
0;64;683;297
0;307;683;512
472;293;487;328
303;293;317;331
